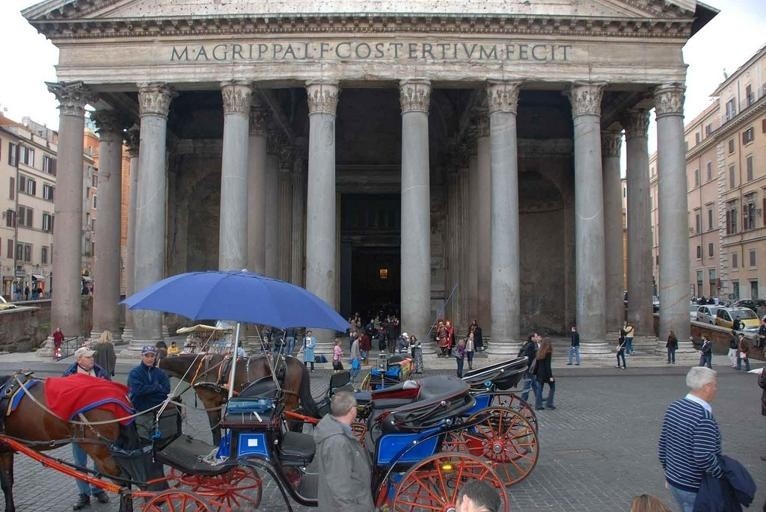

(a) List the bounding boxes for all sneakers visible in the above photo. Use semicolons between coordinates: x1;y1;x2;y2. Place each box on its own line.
566;362;580;366
616;366;625;370
519;397;556;411
73;493;90;510
92;491;109;503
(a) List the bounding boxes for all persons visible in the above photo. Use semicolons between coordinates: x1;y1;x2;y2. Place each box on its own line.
758;349;766;460
303;331;316;374
61;347;111;510
567;326;580;366
311;391;376;512
658;367;728;512
445;478;502;512
56;348;62;362
519;331;556;410
165;326;306;374
348;311;483;378
333;338;344;370
12;280;43;300
80;280;95;295
615;321;634;369
93;330;116;376
690;294;766;371
667;330;679;364
53;328;63;359
127;345;171;447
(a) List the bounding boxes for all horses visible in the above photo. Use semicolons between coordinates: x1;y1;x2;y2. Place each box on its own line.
0;369;137;511
158;350;321;447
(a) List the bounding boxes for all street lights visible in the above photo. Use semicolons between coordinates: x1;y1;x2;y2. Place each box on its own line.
13;140;24;301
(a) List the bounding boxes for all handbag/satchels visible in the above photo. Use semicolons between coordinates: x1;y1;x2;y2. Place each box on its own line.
332;359;339;366
622;330;629;336
451;346;464;360
616;344;622;352
528;357;537;375
739;351;747;358
351;357;361;370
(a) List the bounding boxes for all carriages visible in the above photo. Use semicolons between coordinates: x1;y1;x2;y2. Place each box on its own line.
0;372;509;512
153;344;540;487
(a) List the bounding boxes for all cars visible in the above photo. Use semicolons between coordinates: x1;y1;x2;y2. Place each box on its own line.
0;296;18;313
622;290;766;338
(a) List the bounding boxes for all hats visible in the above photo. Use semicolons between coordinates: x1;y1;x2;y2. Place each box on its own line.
401;332;409;338
141;344;156;356
74;347;96;362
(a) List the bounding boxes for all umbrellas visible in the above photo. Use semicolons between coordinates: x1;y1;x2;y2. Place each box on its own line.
118;269;351;401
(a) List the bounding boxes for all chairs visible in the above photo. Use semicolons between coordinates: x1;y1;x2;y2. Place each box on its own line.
211;358;530;471
